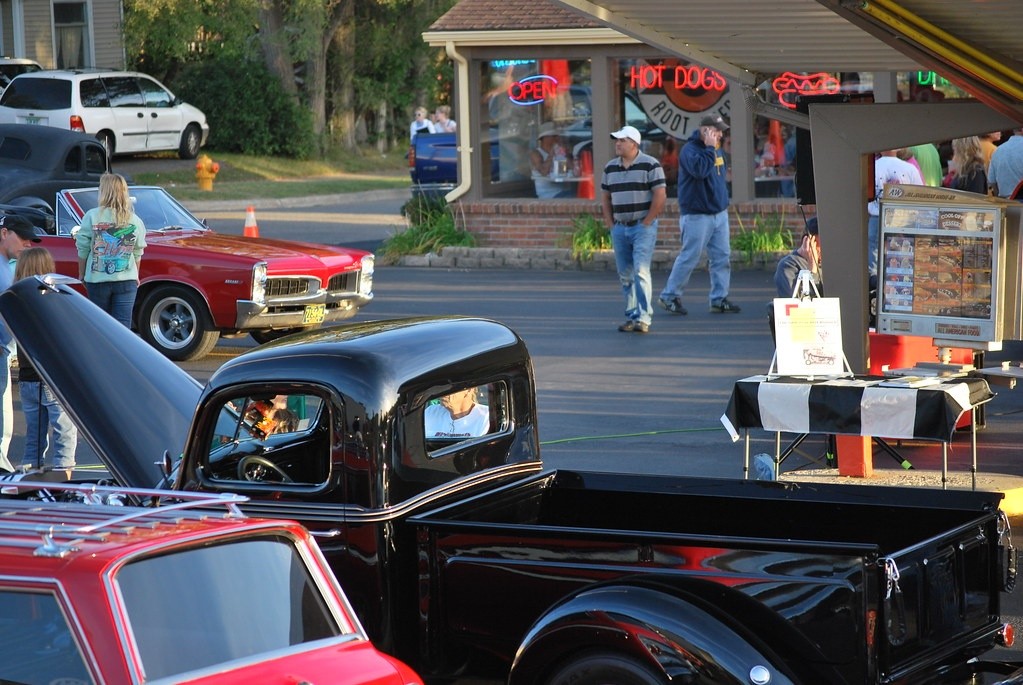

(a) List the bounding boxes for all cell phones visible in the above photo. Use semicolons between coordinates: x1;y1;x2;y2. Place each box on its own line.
703;127;718;142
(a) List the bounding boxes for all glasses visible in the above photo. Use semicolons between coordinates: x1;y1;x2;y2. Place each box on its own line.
414;114;421;116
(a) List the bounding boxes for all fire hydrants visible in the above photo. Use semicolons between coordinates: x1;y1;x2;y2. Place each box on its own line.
195;155;217;191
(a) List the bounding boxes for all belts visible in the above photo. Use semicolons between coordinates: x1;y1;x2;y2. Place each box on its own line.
614;220;639;226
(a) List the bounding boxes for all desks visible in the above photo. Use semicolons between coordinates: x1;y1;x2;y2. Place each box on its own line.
719;371;998;489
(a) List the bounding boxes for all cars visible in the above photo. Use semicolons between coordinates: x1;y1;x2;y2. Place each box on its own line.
0;61;42;95
1;124;121;232
0;186;374;358
0;477;424;685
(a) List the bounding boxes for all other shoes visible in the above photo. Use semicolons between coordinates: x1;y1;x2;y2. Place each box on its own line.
619;321;648;333
657;297;688;315
709;296;741;313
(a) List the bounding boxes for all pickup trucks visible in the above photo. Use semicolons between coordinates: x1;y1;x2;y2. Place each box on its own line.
407;85;663;199
0;272;1023;685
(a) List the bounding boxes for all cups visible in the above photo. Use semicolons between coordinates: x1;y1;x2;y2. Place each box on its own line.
889;240;912;305
559;159;566;176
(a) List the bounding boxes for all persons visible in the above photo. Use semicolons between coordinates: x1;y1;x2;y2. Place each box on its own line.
657;115;741;315
774;217;823;311
530;122;577;198
410;105;456;141
265;408;299;440
75;174;147;330
600;126;667;333
867;125;1023;328
424;387;489;438
0;215;77;473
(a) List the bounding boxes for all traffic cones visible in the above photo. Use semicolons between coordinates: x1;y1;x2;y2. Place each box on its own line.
243;207;261;240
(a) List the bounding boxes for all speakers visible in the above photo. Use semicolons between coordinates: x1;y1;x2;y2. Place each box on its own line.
796;94;875;204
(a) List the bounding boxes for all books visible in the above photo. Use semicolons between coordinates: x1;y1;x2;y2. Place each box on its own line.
879;362;976;388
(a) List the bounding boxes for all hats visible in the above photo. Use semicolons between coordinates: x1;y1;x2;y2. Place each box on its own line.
0;215;42;243
802;215;818;237
699;114;731;131
610;126;641;145
536;122;565;140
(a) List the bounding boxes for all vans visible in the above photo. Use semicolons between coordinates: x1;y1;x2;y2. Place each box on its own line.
0;68;213;161
